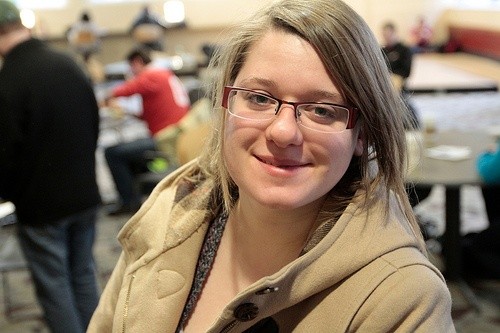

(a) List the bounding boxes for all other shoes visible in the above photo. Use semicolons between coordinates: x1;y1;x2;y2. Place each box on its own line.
108;202;143;217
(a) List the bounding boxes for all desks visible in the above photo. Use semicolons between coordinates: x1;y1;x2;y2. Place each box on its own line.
400;130;500;281
94;113;149;204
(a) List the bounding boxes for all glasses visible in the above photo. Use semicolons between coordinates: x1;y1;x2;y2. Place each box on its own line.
222;85;360;134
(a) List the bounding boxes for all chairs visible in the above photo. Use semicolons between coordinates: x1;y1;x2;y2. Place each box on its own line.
132;98;212;205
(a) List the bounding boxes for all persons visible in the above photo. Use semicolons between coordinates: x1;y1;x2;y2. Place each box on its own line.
0;0;102;333
67;4;500;281
83;0;456;333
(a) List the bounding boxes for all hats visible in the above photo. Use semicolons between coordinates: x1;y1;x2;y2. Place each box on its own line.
0;0;20;24
